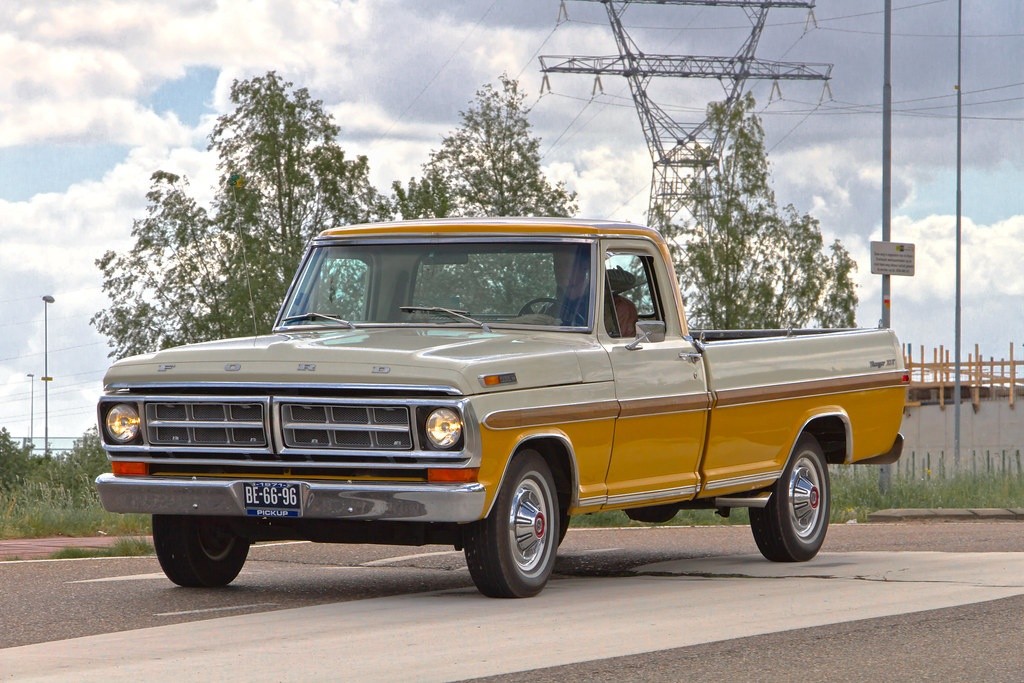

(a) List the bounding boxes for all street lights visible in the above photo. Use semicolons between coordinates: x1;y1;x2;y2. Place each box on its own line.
26;373;34;447
42;295;55;456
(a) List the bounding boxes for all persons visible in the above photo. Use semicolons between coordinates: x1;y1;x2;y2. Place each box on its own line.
539;246;638;338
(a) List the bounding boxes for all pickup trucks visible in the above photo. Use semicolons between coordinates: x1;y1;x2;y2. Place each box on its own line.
94;216;910;599
